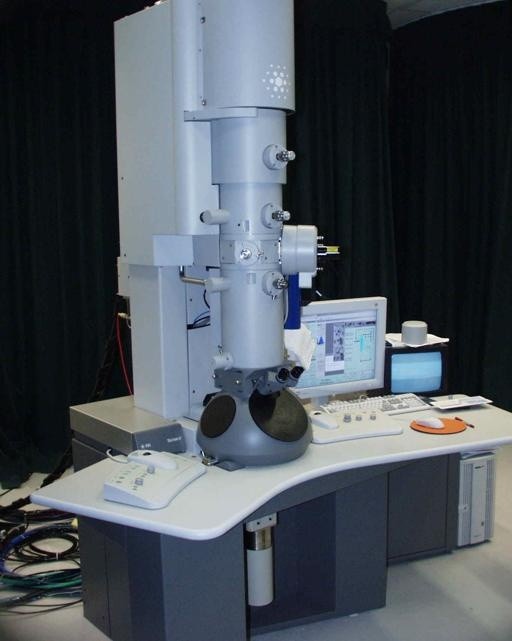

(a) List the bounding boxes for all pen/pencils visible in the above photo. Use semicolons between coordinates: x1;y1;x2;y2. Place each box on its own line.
455;417;474;428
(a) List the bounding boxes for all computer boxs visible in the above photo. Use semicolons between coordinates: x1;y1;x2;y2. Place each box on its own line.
457;452;495;547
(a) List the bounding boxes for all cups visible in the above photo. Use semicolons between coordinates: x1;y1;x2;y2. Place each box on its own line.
400;321;429;346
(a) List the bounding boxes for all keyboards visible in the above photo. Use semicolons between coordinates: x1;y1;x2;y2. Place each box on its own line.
319;392;430;415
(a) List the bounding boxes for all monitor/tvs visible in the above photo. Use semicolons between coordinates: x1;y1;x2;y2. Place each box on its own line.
366;346;450;397
288;296;387;407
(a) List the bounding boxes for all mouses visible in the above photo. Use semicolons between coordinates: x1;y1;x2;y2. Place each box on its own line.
415;415;444;429
